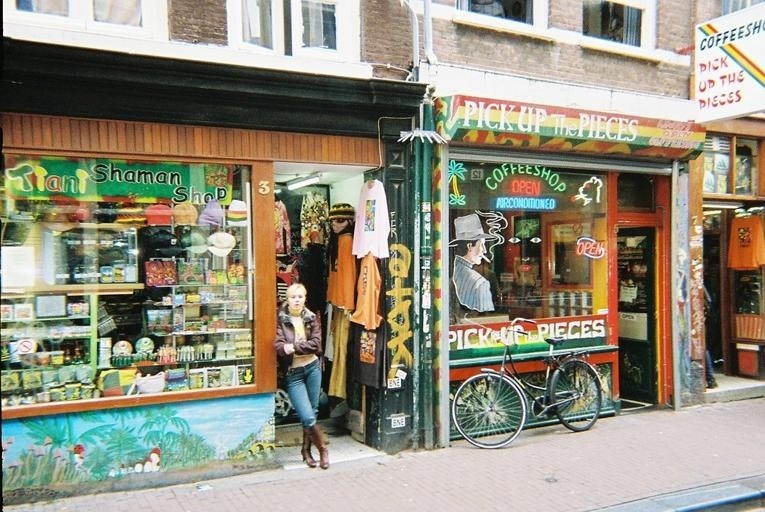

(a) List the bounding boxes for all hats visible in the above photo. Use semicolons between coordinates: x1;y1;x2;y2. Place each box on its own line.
448;213;498;248
328;203;355;221
178;227;209;254
154;235;183;255
198;198;223;227
113;200;148;223
172;200;198;226
92;201;118;222
227;199;248;227
145;204;172;225
207;231;237;257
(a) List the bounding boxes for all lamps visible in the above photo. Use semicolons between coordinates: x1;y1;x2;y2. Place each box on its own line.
286;177;325;192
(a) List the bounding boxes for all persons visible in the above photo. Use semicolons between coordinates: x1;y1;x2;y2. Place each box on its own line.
448;212;497;313
273;282;330;468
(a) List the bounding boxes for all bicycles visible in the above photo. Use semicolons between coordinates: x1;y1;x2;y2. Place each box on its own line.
452;317;607;448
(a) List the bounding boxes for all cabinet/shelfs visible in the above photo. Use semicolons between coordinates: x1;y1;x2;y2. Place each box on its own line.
146;280;252;365
0;289;97;386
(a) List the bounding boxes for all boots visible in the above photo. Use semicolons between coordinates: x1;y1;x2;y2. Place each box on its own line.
303;423;329;470
301;413;318;468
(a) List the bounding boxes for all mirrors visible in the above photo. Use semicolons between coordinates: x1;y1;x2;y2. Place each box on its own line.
546;220;594;290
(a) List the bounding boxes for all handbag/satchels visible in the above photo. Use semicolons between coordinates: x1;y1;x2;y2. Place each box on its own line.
126;371;165;396
166;368;187;391
